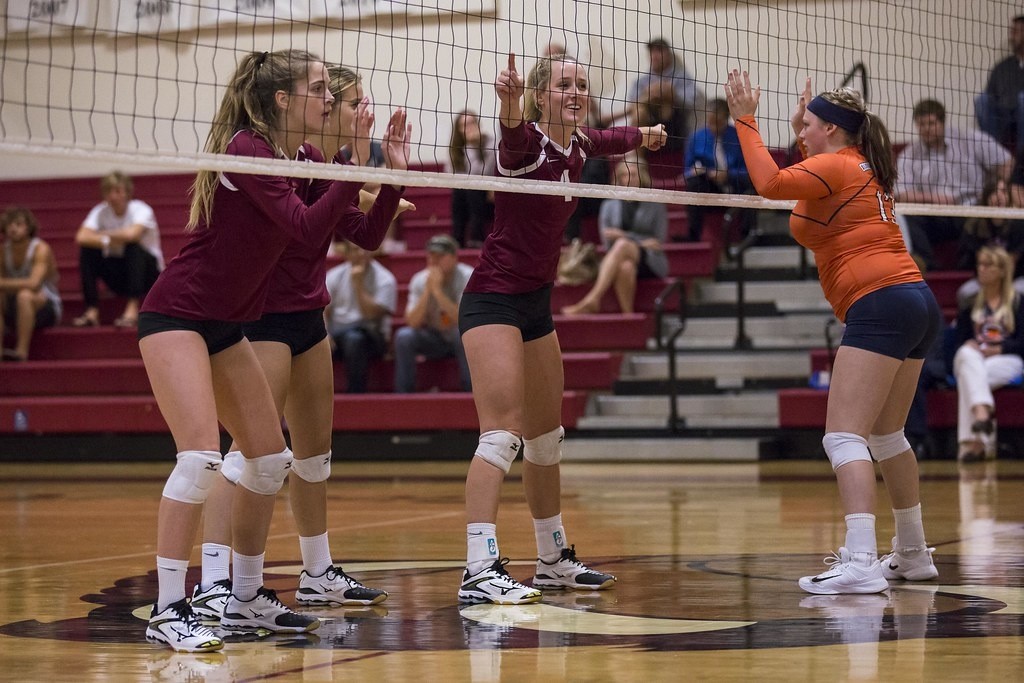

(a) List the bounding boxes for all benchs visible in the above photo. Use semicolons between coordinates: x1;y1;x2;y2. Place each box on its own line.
0;143;1024;459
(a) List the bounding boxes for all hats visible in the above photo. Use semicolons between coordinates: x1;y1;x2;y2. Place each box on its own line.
425;234;458;256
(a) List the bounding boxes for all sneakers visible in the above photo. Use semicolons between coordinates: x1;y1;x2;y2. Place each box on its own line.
880;536;939;580
798;547;888;594
537;587;605;619
457;550;544;602
189;578;233;621
532;543;617;590
145;595;224;652
220;588;321;633
294;565;389;605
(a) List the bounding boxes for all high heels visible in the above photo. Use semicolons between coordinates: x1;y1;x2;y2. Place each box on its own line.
970;416;996;436
959;449;987;464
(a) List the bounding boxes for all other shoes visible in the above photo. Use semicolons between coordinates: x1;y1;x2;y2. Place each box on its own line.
114;318;139;329
73;316;98;326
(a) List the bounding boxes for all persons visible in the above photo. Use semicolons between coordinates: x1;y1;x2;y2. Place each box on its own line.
946;246;1024;461
457;53;666;605
561;157;667;315
895;15;1024;259
135;50;412;650
323;238;397;391
72;170;166;327
724;69;942;594
0;207;62;360
394;235;475;393
443;38;754;250
187;57;416;621
327;119;407;256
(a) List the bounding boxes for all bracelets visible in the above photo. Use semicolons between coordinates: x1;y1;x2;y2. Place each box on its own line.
101;235;112;258
